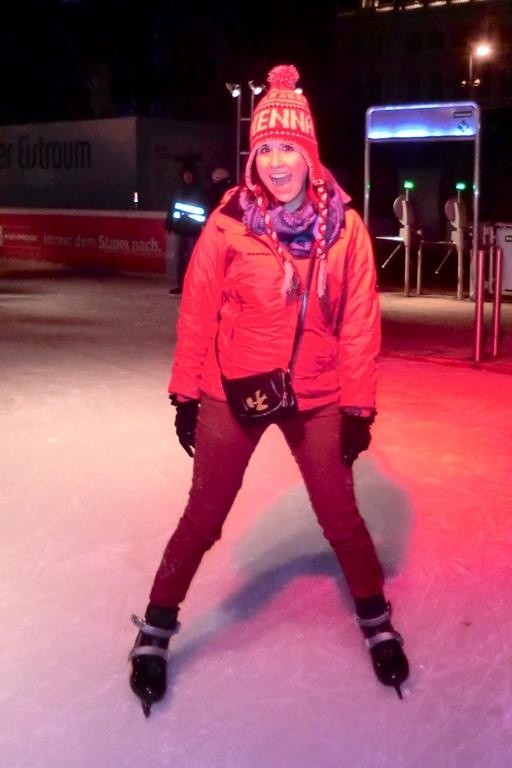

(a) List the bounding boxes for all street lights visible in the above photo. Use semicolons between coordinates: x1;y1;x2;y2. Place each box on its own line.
460;43;492;99
225;79;267;186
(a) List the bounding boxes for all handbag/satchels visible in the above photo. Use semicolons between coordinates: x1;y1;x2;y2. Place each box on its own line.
221;368;296;427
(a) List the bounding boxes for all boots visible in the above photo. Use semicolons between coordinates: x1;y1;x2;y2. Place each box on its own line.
354;591;409;685
128;603;181;702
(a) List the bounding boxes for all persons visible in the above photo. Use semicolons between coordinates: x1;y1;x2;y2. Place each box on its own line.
126;63;413;724
209;166;236;206
164;158;210;297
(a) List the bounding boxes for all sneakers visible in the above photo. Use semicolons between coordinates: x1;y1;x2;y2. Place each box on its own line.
169;287;182;294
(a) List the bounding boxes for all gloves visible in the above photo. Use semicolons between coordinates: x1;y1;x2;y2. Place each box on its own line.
339;408;378;466
170;394;201;457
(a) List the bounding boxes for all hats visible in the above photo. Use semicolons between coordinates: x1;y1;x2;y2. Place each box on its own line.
245;64;325;192
212;167;231;183
